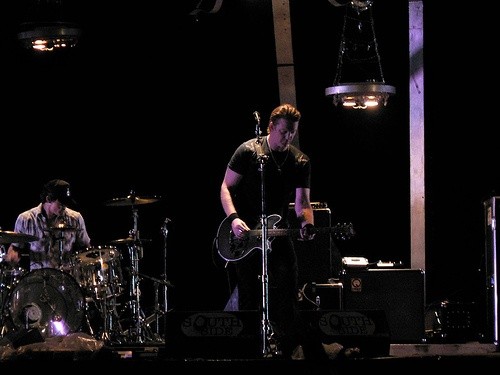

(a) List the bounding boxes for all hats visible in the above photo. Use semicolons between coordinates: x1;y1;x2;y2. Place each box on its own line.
46;179;77;206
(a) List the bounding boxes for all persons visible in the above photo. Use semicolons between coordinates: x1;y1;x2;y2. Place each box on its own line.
220;104;329;359
7;180;92;271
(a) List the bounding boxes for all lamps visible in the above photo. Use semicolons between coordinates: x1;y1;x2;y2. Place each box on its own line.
325;0;396;110
16;0;80;51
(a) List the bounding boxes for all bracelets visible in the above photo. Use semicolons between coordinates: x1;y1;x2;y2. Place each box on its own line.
228;212;239;224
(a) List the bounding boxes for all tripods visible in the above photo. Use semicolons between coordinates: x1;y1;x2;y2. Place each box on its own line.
83;207;168;345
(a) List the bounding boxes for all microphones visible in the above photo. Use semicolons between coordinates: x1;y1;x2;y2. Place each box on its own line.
253;111;260;125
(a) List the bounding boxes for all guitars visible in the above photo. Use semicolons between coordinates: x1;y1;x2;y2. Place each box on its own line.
217;214;354;267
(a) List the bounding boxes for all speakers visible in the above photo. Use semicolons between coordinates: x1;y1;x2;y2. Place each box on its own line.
165;311;263;359
287;207;332;308
344;267;425;343
282;309;390;358
482;196;500;343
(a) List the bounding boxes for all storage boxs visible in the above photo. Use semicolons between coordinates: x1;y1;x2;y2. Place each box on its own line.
339;269;426;344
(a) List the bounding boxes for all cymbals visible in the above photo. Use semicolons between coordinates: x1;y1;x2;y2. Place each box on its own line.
0;230;39;243
108;197;160;206
41;226;83;232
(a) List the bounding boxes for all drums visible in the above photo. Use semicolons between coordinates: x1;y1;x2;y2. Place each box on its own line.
6;268;85;342
71;245;127;301
